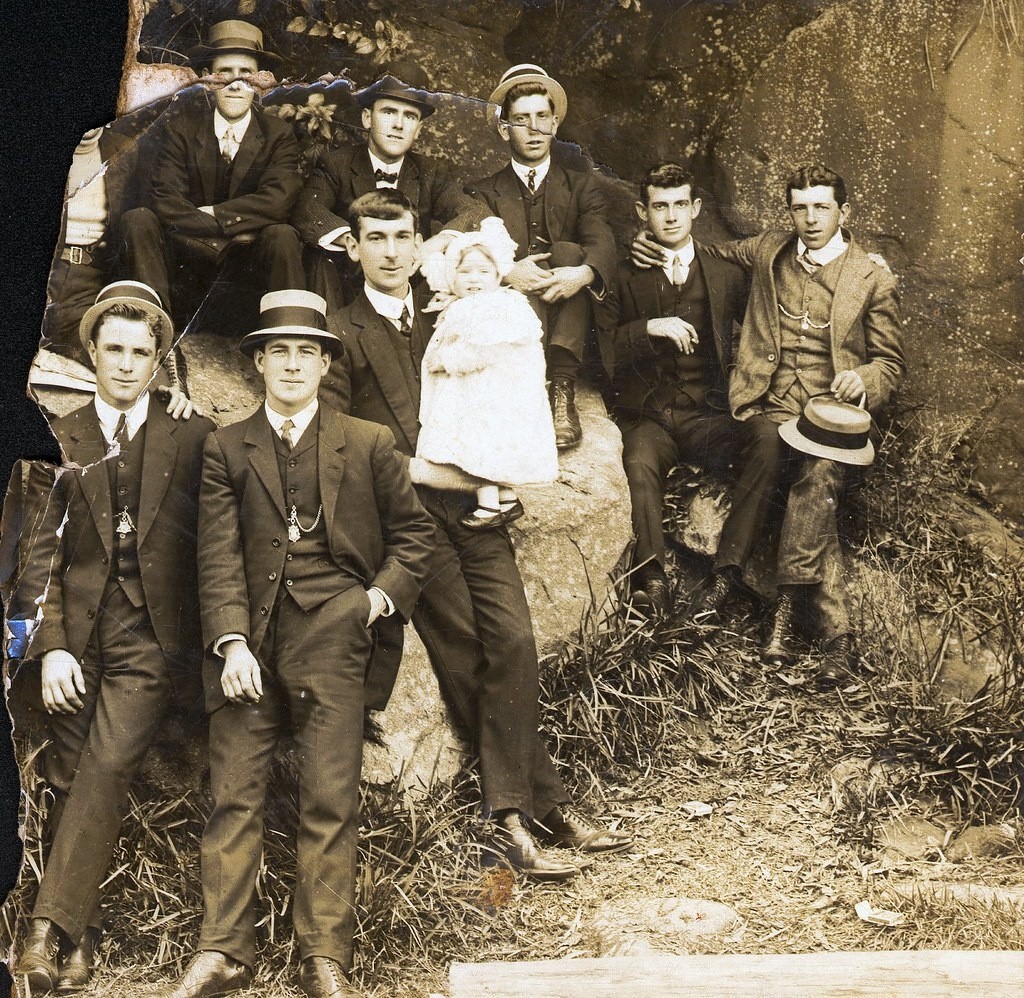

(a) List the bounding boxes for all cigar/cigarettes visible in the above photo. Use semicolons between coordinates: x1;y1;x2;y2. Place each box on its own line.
690;337;699;344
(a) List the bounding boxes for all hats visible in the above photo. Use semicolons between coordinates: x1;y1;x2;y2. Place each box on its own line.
239;289;345;360
79;281;174;358
777;397;875;465
355;62;435;121
486;63;567;136
187;20;284;77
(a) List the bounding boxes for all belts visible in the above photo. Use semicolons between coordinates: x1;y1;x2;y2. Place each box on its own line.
62;246;92;266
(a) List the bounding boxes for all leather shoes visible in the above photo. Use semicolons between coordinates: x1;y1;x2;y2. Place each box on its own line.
160;342;190;401
815;634;858;687
549;378;584;449
696;572;739;623
533;803;635;856
141;950;251;998
628;578;669;621
295;956;365;998
759;601;799;666
56;927;102;990
15;918;60;993
476;808;580;880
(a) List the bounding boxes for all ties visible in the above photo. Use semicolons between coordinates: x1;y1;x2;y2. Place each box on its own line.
280;420;296;453
222;128;234;166
672;255;684;292
524;170;536;191
112;413;130;451
398;303;412;341
803;249;818;266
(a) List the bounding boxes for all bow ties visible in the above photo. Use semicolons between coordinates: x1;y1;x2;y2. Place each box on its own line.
374;169;398;184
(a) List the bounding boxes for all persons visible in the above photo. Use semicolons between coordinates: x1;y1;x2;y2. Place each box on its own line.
43;121;140;367
462;64;616;449
120;19;307;400
296;62;495;312
0;280;214;993
417;218;557;530
141;289;435;998
632;166;902;684
321;190;637;880
617;162;891;642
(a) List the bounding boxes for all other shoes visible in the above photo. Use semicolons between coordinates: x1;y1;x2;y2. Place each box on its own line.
500;498;524;525
461;505;504;530
